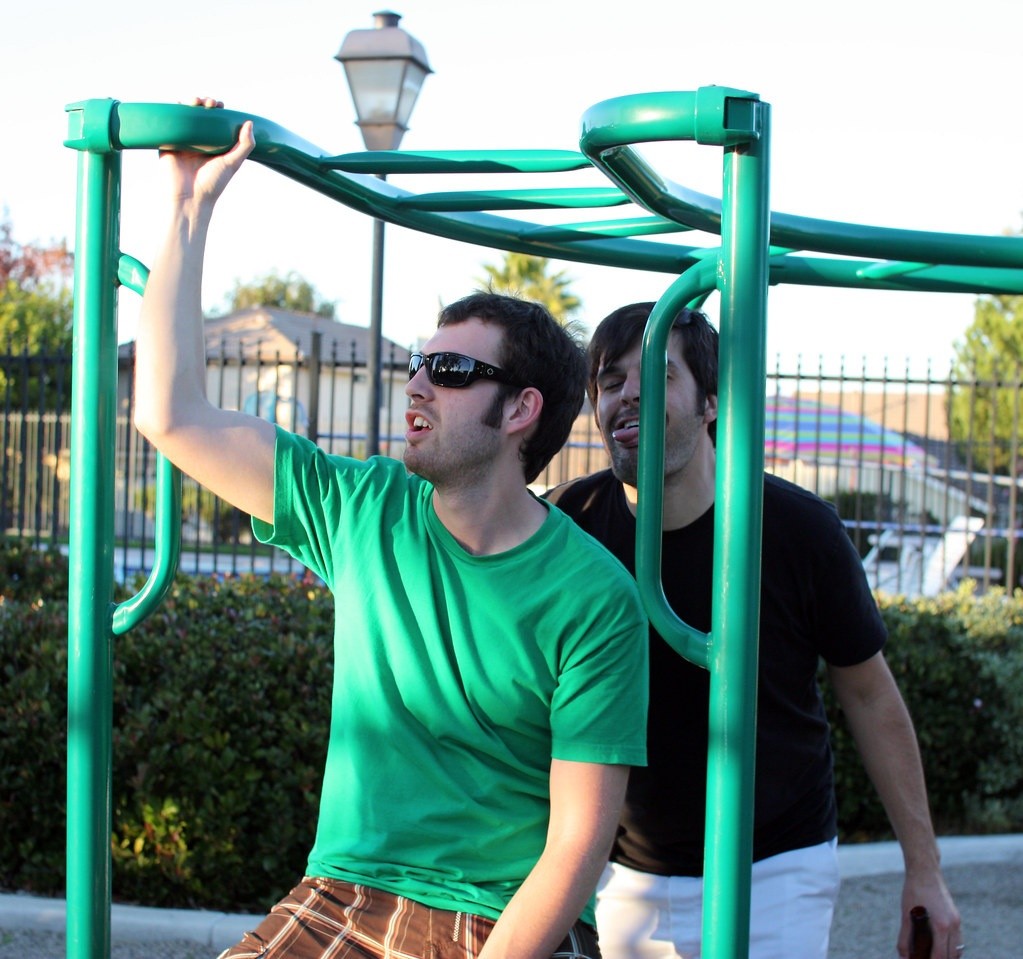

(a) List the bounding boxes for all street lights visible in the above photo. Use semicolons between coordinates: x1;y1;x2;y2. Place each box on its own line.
336;10;433;450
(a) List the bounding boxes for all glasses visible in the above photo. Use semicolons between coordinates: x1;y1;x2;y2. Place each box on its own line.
408;353;508;389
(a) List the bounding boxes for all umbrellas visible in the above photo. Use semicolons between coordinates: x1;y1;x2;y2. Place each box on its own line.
765;394;942;486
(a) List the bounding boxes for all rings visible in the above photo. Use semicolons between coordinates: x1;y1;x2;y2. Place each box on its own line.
956;944;965;950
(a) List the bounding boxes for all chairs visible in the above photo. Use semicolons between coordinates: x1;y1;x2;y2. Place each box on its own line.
859;516;984;599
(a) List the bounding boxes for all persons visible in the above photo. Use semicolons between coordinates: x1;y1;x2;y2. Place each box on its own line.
538;302;967;959
131;97;649;959
